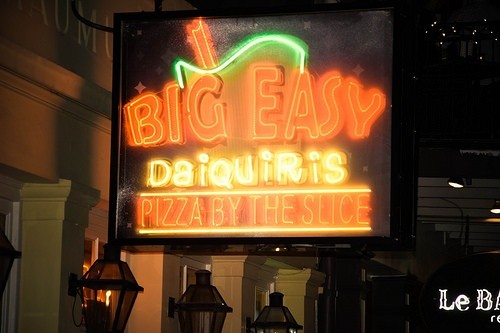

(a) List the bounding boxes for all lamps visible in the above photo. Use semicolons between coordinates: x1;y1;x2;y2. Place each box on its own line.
489;199;500;214
447;176;464;189
